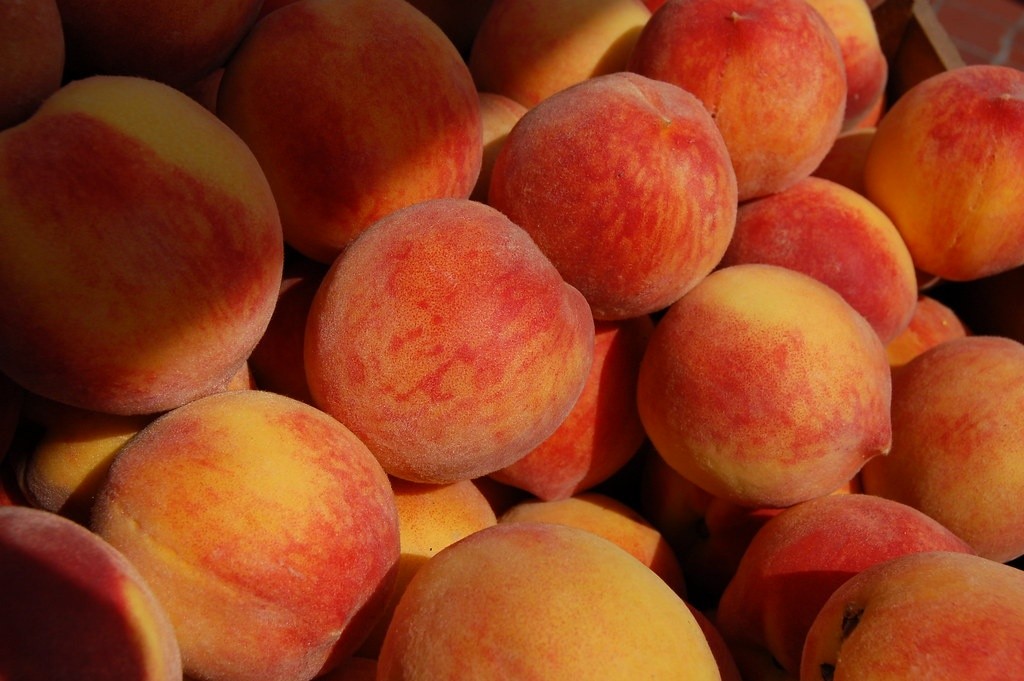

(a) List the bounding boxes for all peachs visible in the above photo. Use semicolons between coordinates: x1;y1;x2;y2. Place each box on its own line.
0;0;1024;681
